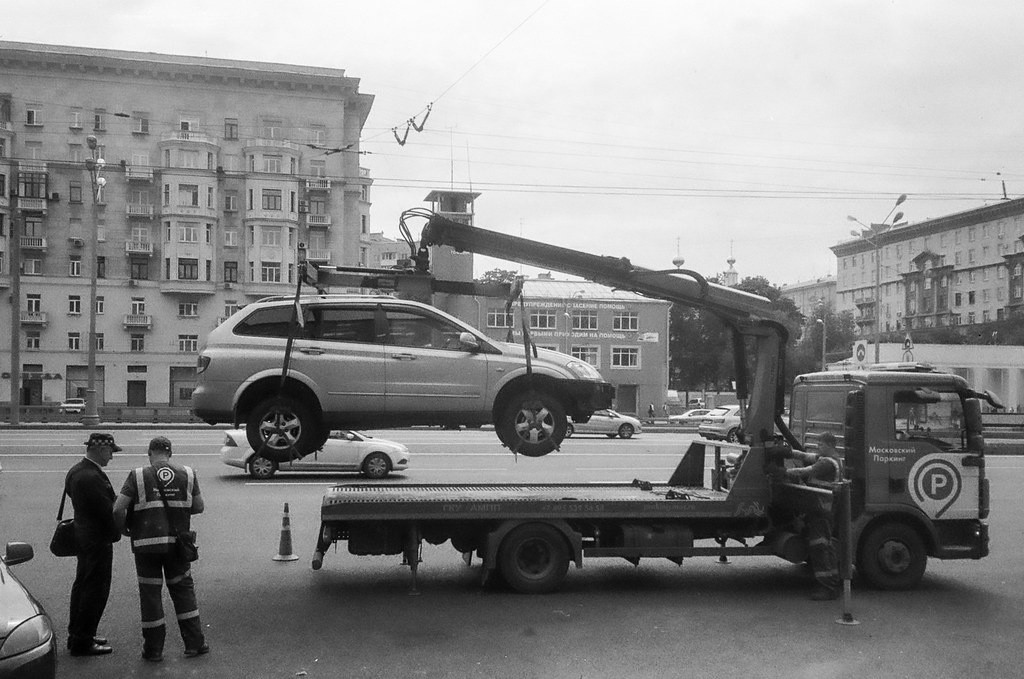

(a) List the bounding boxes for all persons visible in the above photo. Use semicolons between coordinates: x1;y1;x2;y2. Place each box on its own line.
662;401;667;416
929;411;944;425
648;402;656;418
113;436;209;662
952;408;959;424
909;407;915;425
783;432;842;601
65;432;123;658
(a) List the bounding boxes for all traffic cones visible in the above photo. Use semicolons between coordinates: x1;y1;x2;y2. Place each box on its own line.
272;503;299;561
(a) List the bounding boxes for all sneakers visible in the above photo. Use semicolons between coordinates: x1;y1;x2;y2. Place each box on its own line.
185;643;210;657
142;649;164;662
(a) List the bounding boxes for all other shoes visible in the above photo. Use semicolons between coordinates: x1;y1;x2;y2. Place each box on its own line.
811;590;835;600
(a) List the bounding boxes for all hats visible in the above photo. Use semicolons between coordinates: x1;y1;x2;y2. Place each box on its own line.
84;434;121;451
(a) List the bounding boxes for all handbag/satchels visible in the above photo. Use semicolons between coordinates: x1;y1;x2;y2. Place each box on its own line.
176;531;198;562
50;519;79;556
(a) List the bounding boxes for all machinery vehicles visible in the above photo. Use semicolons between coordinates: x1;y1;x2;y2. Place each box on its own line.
249;208;1005;625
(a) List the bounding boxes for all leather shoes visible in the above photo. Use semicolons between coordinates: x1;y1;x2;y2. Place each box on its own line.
92;636;107;645
85;642;112;655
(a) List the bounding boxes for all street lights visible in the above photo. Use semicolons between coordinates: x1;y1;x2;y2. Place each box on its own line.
847;193;907;363
563;289;586;355
81;135;107;425
816;298;827;372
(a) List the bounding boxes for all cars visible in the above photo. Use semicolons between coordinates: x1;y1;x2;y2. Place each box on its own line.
0;541;57;679
668;408;711;424
220;423;410;479
564;408;643;439
59;398;86;414
688;399;704;409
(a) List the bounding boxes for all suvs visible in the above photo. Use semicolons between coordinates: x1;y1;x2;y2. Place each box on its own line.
191;293;616;463
698;405;790;444
